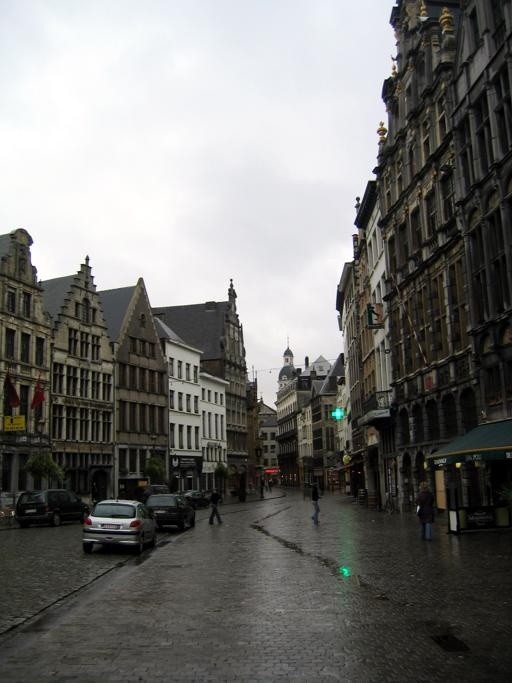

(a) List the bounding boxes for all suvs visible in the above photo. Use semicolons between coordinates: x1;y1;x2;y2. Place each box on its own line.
15;490;89;526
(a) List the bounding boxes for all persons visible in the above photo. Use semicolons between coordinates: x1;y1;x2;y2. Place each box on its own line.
209;488;223;525
415;482;435;540
310;483;322;528
260;476;272;499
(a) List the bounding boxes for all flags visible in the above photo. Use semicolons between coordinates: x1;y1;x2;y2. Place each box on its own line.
32;381;45;406
4;373;20;408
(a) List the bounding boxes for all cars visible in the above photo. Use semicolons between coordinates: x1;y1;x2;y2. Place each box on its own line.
81;500;156;553
183;490;212;510
147;494;196;529
